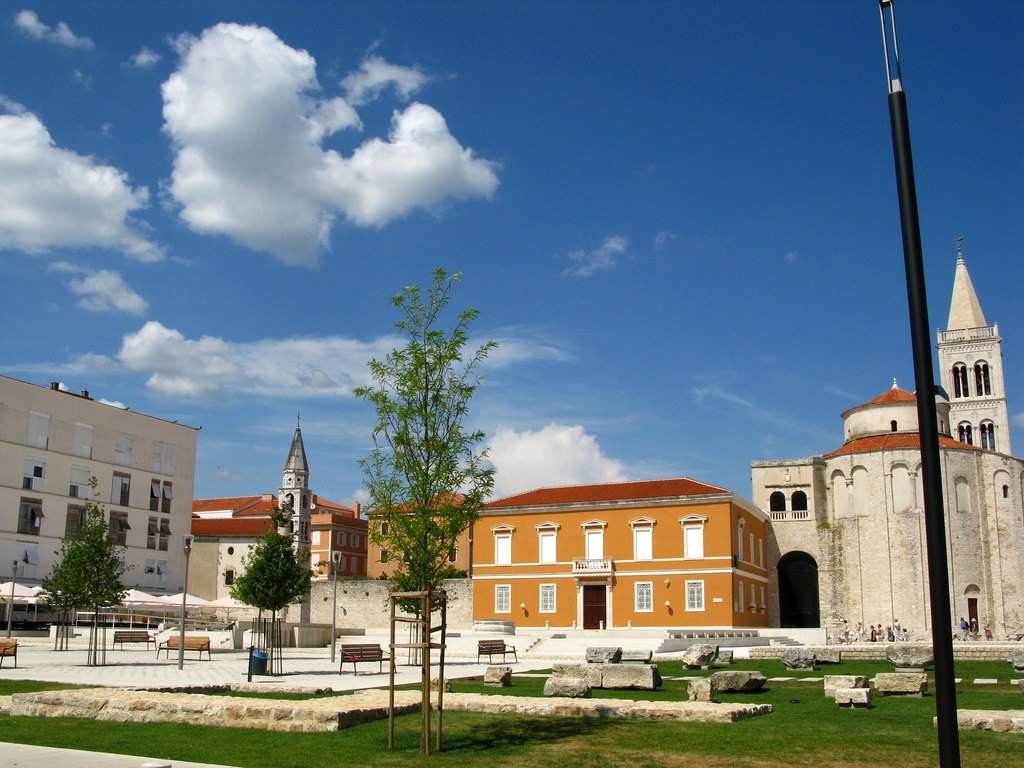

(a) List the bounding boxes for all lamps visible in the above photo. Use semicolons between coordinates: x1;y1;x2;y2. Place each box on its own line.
521;603;526;609
665;601;671;607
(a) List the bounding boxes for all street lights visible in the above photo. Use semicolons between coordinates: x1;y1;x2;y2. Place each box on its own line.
331;551;343;663
7;559;19;639
177;533;195;671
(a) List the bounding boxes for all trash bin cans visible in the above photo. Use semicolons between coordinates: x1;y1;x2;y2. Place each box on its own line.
252;651;268;675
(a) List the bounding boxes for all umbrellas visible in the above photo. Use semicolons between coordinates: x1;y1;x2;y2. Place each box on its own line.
0;581;252;629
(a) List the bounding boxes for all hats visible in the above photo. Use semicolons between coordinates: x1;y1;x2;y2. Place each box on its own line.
971;618;976;622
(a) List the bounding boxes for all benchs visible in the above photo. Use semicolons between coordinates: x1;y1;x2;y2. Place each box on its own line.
113;631;158;651
0;637;19;668
339;644;397;676
156;636;211;661
477;639;518;666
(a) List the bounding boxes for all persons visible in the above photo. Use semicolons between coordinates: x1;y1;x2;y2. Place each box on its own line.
960;617;991;641
843;618;909;645
